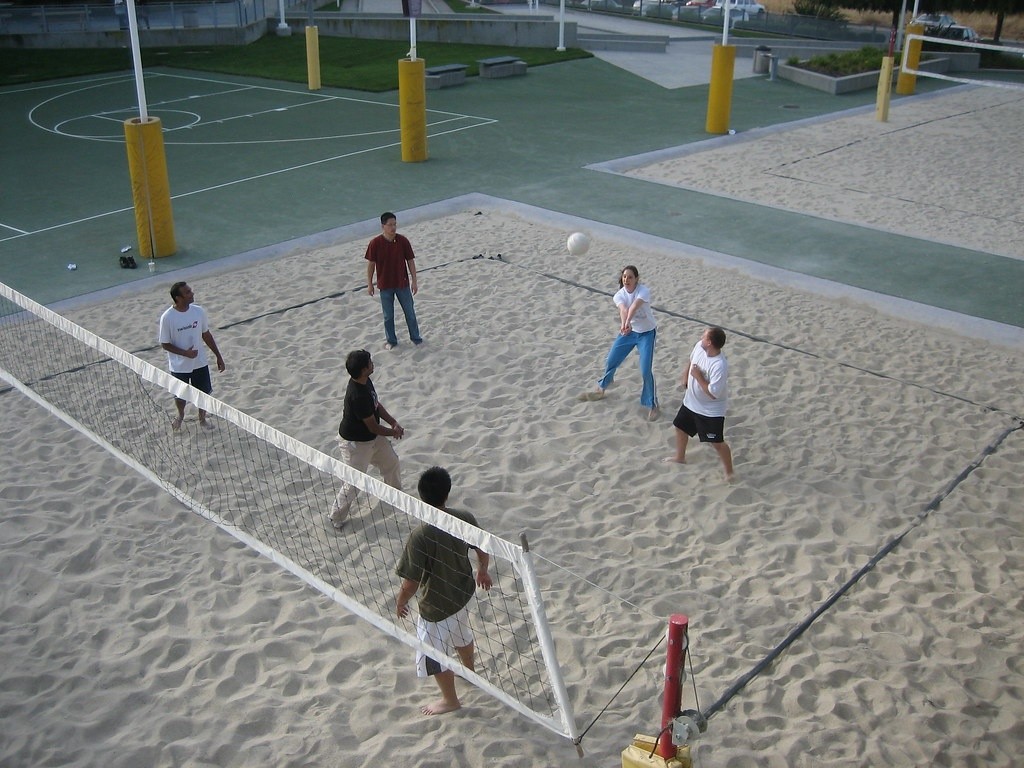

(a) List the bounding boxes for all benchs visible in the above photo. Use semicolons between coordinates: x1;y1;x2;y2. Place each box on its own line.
475;56;528;79
426;63;470;89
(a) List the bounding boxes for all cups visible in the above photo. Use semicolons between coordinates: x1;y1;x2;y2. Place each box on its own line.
148;262;156;272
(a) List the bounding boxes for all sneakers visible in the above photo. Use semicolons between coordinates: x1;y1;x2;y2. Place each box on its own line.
119;257;128;268
126;256;136;268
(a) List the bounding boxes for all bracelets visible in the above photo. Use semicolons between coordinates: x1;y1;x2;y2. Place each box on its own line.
697;375;703;381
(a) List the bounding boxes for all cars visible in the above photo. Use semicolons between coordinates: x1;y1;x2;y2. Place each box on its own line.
579;0;766;30
911;13;954;34
939;25;979;43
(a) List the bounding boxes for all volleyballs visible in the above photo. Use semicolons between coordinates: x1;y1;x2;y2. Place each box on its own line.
566;232;590;256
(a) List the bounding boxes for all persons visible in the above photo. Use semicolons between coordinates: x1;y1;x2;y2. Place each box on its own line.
673;327;733;478
365;212;422;349
159;282;225;431
327;349;404;530
597;265;659;417
115;0;129;30
134;0;150;29
395;465;493;716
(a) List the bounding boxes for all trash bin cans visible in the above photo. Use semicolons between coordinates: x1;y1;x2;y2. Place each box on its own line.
753;46;772;74
